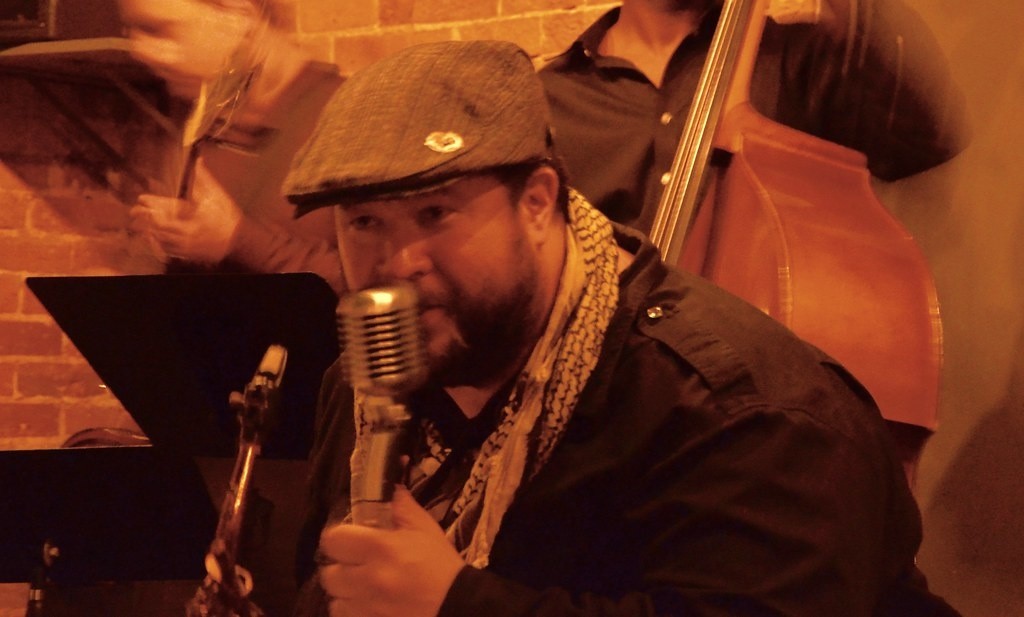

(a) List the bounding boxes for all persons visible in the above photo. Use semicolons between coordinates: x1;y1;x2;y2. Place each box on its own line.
121;0;350;294
536;0;976;259
280;39;965;617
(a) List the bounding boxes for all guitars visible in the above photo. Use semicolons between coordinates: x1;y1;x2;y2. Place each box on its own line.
159;27;267;272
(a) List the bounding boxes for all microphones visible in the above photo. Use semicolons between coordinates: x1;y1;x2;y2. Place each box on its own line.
335;281;430;534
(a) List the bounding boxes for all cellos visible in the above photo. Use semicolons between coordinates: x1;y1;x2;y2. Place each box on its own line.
633;0;947;491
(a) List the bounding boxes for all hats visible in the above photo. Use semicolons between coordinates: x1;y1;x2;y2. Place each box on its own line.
279;39;554;219
